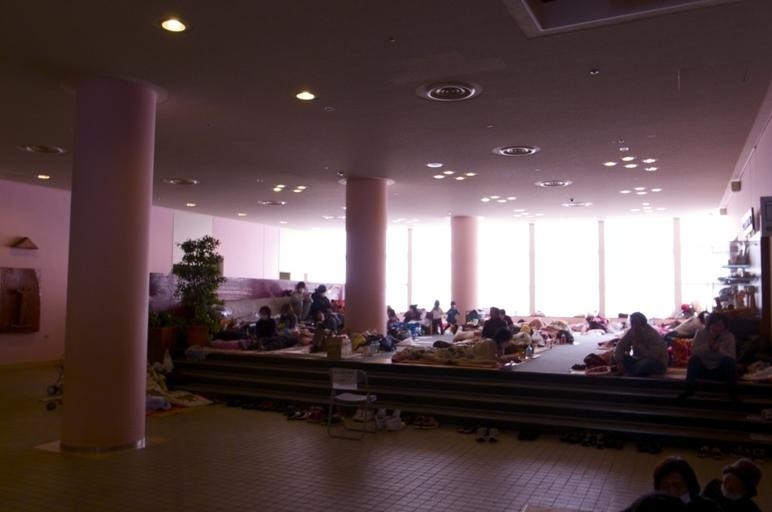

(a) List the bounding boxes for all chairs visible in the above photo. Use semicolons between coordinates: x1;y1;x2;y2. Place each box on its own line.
326;368;376;441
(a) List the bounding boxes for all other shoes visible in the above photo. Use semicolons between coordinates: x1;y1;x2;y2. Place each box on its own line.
562;429;663;454
457;426;541;443
213;394;439;430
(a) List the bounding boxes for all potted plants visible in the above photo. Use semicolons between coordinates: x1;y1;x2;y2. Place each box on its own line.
172;238;226;348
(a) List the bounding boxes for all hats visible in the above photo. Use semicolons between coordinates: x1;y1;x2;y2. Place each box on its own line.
722;456;763;497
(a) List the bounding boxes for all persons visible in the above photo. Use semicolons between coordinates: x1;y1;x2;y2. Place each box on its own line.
255;282;525;363
614;312;669;377
678;312;738;401
619;455;763;511
